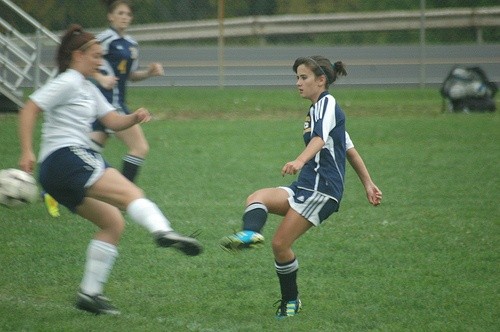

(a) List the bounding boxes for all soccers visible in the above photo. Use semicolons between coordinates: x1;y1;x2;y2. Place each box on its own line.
0;168;37;208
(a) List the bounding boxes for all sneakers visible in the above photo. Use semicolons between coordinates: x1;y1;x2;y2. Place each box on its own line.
75;291;121;315
155;231;201;256
272;298;302;320
40;189;61;218
220;230;265;252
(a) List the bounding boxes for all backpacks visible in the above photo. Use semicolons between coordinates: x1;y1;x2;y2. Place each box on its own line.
440;64;497;114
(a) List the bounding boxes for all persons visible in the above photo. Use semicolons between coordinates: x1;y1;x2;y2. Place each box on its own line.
219;55;382;323
18;23;202;316
41;0;164;218
